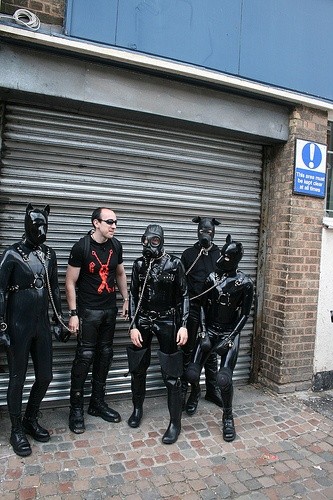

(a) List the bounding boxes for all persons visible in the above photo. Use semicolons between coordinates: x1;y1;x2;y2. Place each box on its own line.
182;234;253;442
65;207;130;434
125;225;189;444
0;201;67;457
178;216;224;408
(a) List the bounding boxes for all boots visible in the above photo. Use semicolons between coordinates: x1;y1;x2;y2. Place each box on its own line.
87;379;121;423
124;344;151;427
157;351;182;444
69;389;85;434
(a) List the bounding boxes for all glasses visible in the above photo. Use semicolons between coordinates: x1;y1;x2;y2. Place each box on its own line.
98;220;118;225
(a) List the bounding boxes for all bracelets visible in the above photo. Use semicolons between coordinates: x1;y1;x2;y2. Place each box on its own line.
122;297;129;302
66;309;77;317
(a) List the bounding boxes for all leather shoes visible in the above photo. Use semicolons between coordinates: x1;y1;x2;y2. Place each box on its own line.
10;427;32;455
222;407;236;441
22;418;50;442
205;382;224;407
181;383;201;415
